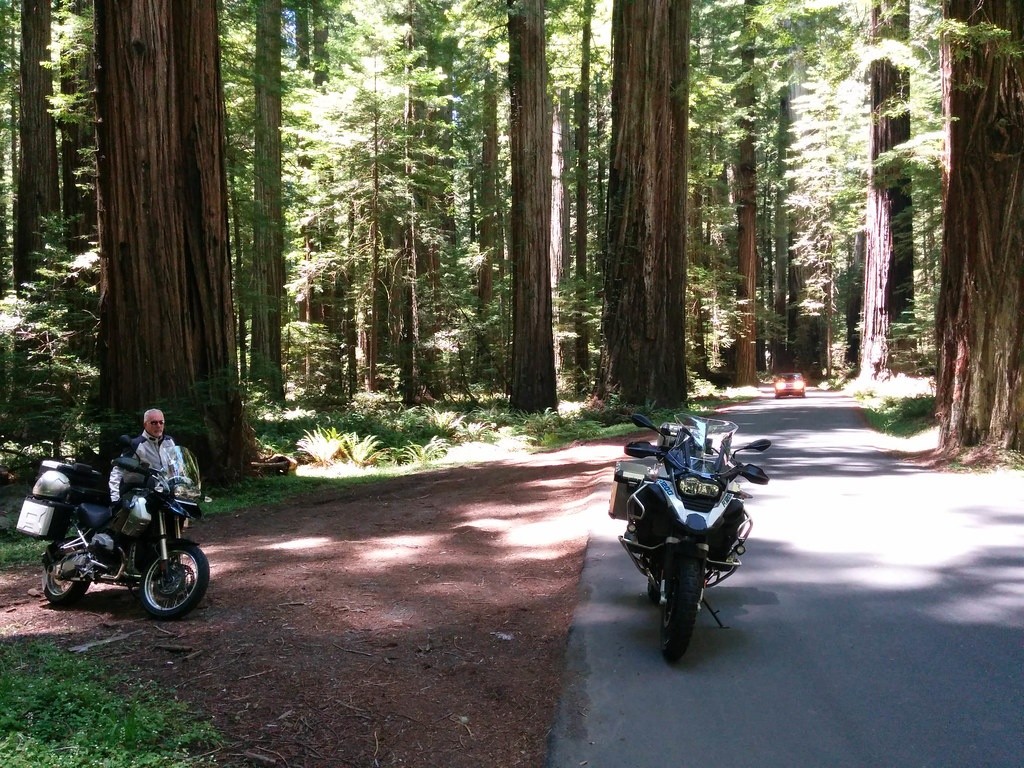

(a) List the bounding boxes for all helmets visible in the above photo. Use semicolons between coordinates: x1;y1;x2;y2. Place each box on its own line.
32;470;70;498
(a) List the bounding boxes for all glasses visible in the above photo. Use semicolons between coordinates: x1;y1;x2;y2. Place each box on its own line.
151;420;164;425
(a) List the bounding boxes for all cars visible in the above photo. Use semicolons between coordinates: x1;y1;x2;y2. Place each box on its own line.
774;373;810;401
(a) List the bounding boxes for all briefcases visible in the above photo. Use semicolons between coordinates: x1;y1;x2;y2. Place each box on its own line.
16;496;73;538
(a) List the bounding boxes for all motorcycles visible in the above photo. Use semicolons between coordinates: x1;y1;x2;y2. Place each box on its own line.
611;410;772;663
16;436;213;620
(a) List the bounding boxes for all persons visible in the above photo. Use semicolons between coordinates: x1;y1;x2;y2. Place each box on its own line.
108;408;178;597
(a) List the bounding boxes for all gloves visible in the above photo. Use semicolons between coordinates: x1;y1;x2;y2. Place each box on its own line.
112;501;122;517
